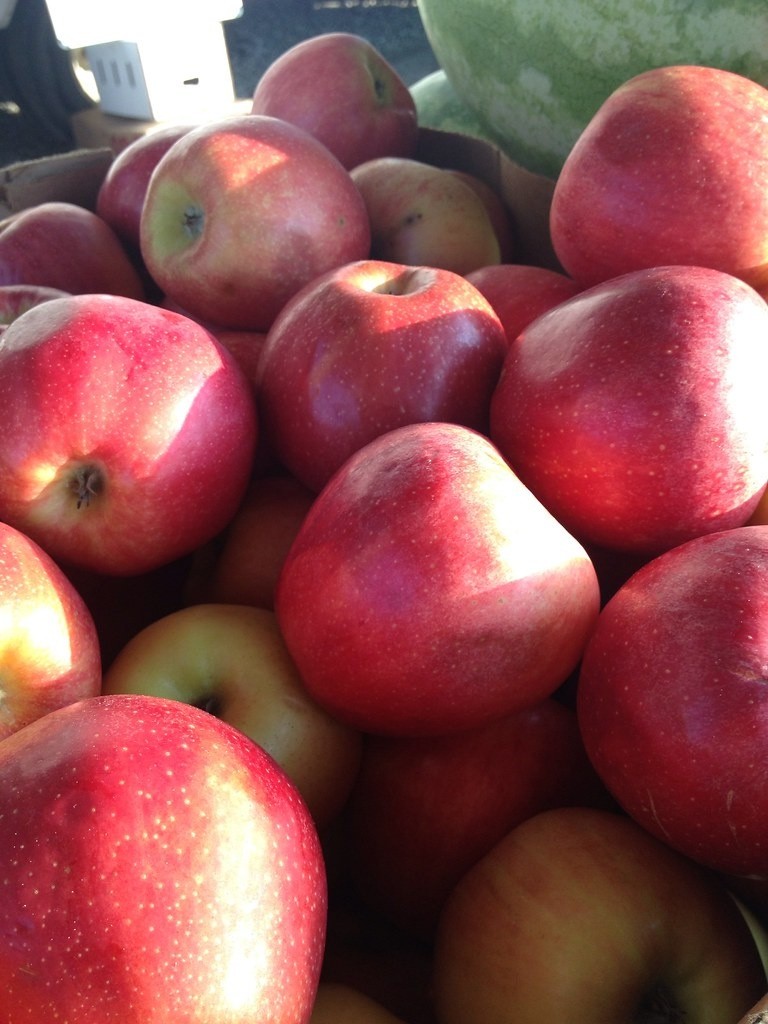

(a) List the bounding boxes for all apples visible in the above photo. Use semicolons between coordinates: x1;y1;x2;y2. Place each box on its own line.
0;31;768;1024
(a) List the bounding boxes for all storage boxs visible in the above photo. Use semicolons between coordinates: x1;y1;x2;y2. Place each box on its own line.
0;125;566;275
70;104;160;154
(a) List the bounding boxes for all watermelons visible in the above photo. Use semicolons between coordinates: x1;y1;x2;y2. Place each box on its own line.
385;0;768;186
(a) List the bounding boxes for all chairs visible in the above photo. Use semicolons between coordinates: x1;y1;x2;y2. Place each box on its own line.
84;40;158;122
